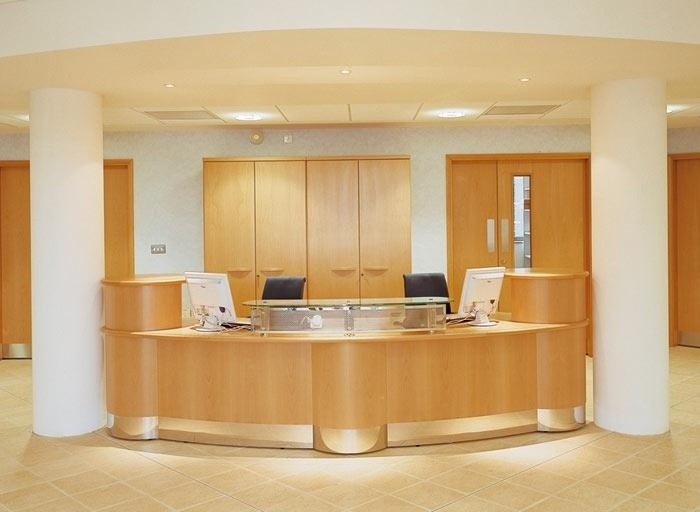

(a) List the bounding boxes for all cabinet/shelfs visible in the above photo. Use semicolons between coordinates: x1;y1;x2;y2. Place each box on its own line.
307;154;411;300
202;156;306;318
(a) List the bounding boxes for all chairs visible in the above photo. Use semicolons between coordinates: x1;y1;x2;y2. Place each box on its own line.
403;272;452;316
260;276;306;300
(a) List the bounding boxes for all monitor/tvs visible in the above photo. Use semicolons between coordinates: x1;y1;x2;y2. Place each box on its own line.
185;271;237;332
458;266;506;327
